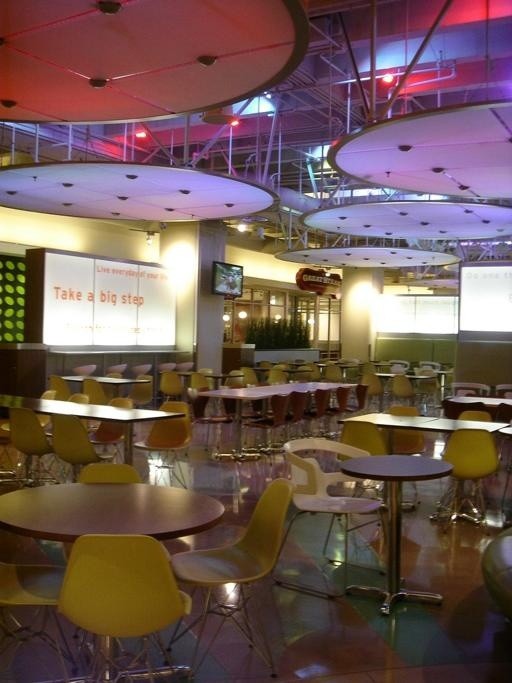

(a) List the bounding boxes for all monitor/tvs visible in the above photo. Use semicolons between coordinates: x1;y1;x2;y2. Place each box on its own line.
212;261;243;297
